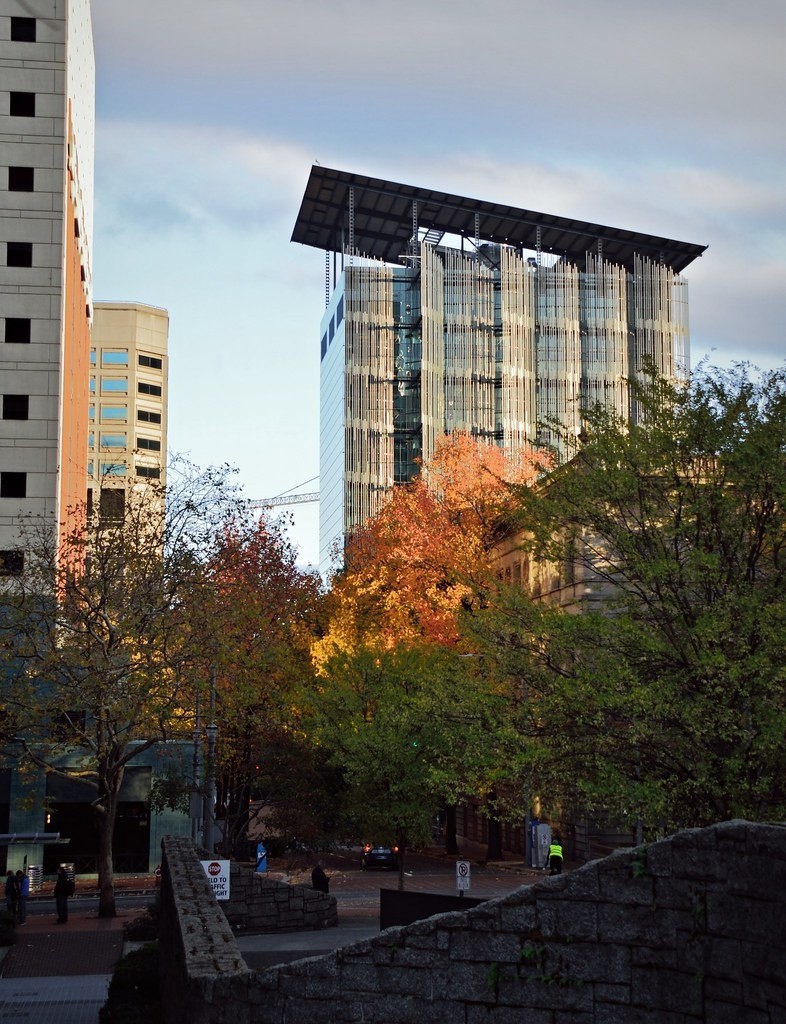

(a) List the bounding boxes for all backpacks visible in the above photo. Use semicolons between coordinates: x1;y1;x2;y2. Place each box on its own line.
67;878;74;896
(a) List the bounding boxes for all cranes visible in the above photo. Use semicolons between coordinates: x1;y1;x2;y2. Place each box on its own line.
226;475;321;510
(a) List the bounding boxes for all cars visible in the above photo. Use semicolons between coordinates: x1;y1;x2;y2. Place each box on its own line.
362;834;401;867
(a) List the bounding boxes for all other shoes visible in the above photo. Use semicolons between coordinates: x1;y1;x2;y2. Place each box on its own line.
16;922;26;927
51;921;67;925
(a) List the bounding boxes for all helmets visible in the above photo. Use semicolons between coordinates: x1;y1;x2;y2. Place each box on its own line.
552;840;558;845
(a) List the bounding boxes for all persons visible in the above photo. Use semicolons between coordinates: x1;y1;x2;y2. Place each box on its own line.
311;860;330;894
545;840;562;874
4;870;29;925
54;867;70;923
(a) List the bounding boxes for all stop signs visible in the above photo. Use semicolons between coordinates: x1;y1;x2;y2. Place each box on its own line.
208;862;221;875
